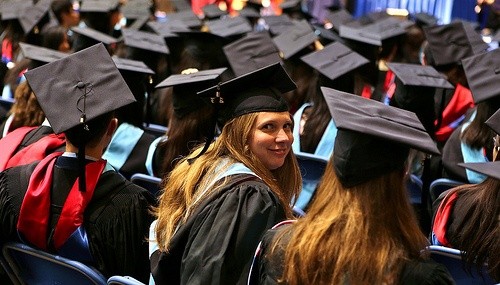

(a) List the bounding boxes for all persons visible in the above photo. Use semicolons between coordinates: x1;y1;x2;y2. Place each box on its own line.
431;161;500;272
0;0;500;185
148;61;304;285
0;40;156;285
237;85;458;285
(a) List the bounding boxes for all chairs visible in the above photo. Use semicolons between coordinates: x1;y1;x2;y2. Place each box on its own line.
0;98;500;285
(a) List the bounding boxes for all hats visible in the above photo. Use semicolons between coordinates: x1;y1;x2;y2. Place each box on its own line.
485;108;500;135
155;68;229;119
461;47;500;105
187;61;298;165
456;161;500;180
23;42;137;192
385;61;455;130
113;55;155;127
320;85;443;216
423;22;475;67
299;42;370;81
1;0;499;46
222;30;283;77
18;42;69;62
